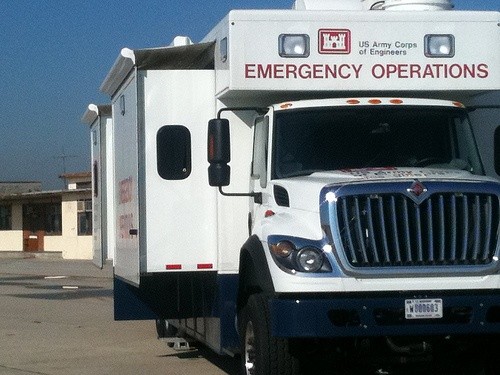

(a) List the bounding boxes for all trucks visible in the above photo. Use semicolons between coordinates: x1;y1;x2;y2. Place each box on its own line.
80;0;500;375
